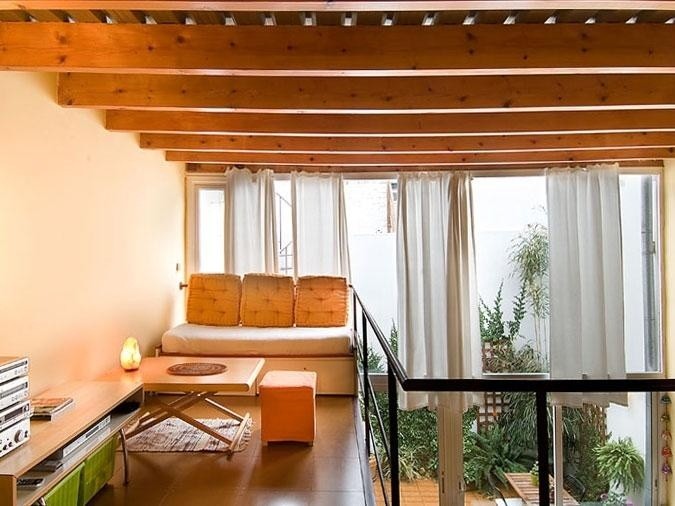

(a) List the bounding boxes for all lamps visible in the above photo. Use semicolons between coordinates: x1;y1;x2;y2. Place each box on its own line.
120;337;142;372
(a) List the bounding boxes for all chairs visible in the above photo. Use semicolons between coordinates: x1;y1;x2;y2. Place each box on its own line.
492;486;526;506
563;472;586;502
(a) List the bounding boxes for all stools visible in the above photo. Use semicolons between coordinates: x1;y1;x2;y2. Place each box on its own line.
258;370;317;447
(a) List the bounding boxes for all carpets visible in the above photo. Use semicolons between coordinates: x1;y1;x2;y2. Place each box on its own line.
115;415;254;453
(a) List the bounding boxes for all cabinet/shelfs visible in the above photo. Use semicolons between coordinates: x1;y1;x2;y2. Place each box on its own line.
0;378;145;506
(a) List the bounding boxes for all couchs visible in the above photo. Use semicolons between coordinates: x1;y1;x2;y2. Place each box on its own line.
149;281;357;396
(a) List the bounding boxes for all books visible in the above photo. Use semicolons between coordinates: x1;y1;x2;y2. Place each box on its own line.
30;396;75;421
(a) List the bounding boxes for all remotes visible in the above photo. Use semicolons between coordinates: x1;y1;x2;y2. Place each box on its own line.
16;478;45;488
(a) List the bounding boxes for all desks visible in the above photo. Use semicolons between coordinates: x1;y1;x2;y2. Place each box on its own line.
503;471;580;506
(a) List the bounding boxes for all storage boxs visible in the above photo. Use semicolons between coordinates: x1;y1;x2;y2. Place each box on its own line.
31;461;86;506
78;436;116;506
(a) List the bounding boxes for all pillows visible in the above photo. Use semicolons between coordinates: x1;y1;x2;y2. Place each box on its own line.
239;272;295;327
293;274;349;327
185;273;242;325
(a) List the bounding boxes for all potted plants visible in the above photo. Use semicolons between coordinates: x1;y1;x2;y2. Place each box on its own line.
529;461;539;485
